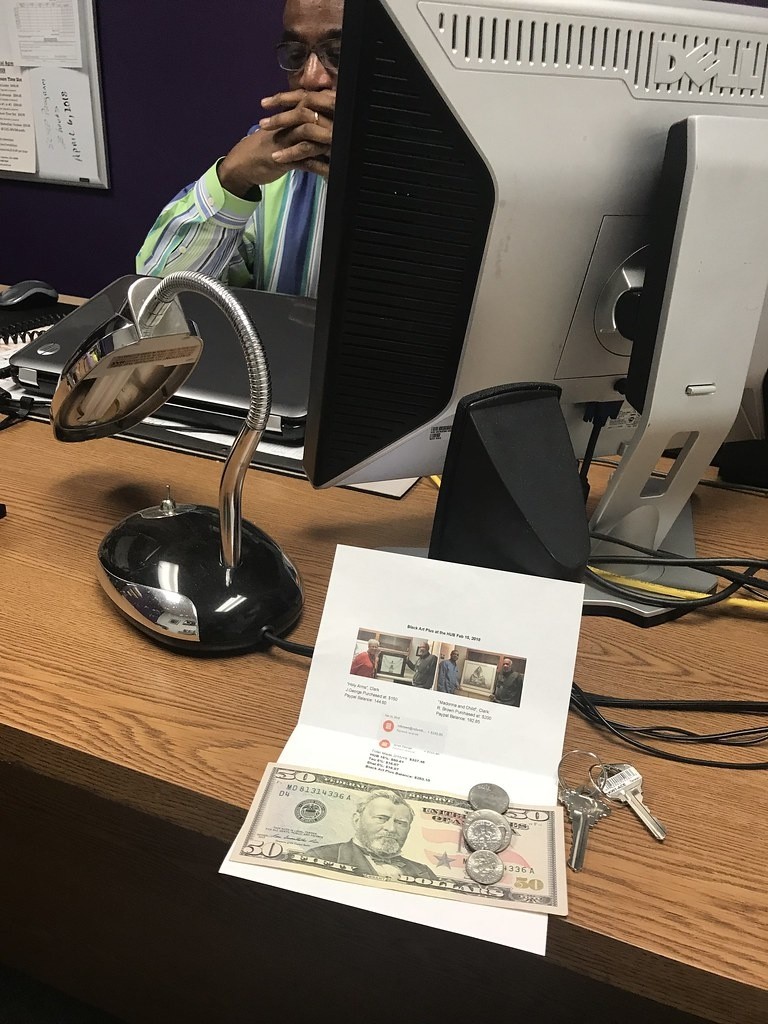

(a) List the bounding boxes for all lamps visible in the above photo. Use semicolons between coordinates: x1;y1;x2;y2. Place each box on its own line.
46;272;305;663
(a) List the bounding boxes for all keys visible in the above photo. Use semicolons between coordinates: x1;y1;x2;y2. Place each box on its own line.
597;763;667;842
558;785;612;874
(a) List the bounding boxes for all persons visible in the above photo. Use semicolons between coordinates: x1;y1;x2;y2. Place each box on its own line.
351;639;379;678
490;659;522;707
437;650;460;694
135;1;346;298
405;642;438;689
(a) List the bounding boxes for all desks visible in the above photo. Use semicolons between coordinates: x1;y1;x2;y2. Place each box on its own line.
1;286;767;1017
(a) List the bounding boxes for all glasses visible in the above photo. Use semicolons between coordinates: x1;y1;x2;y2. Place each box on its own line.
273;37;342;70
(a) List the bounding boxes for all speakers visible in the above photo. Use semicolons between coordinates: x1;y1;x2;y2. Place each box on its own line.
424;379;589;588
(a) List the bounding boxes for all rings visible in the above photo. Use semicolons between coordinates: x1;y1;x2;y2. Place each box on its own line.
314;111;318;125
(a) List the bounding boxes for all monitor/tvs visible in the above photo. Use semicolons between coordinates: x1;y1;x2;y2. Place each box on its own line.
301;0;768;492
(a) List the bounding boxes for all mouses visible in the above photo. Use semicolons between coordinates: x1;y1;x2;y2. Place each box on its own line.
3;281;55;308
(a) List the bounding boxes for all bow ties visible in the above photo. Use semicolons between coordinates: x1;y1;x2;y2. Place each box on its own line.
369;853;407;869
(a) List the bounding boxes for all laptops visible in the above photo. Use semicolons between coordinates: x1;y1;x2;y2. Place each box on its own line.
13;276;316;448
